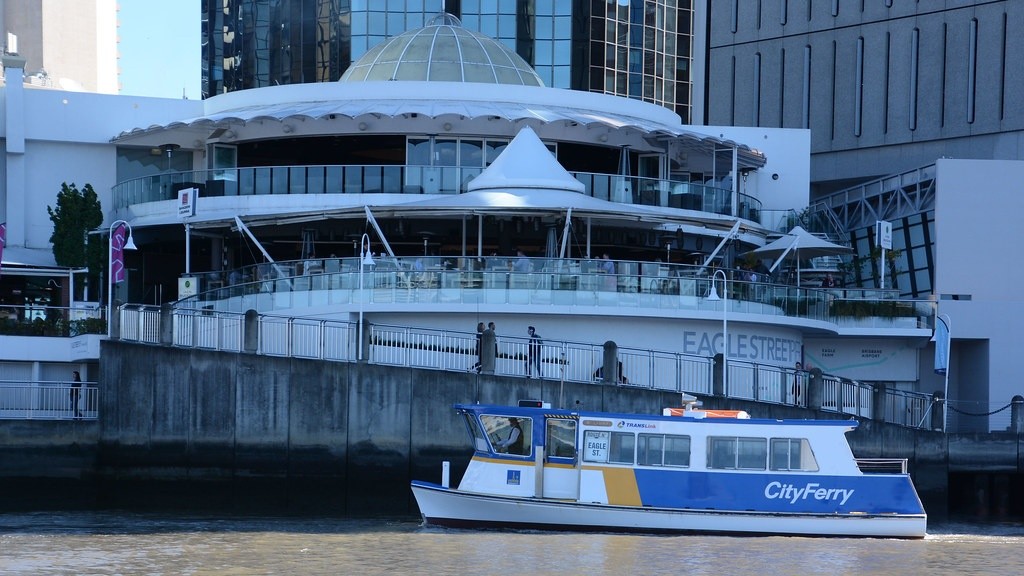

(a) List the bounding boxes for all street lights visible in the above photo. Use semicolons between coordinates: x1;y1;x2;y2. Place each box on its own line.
358;234;377;362
106;220;138;337
706;269;729;398
929;312;951;435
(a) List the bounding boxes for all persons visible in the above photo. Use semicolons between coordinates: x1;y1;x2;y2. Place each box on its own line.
488;250;530;288
792;362;812;407
581;254;614;288
525;326;543;379
822;272;837;316
303;252;315;275
467;322;499;374
494;416;524;455
416;257;424;270
437;259;453;288
740;267;757;300
70;371;83;420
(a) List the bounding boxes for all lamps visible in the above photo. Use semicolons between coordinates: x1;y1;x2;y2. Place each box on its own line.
681;393;697;411
682;399;703;411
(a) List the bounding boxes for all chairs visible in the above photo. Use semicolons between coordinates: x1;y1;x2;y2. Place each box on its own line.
207;254;779;305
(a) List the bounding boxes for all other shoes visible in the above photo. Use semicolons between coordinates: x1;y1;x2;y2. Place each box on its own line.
79;413;82;420
476;368;479;374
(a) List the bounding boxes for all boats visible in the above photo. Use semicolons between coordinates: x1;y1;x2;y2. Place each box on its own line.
411;397;928;542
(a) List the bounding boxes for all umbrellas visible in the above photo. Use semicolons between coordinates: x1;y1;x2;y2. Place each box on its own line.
738;225;855;316
